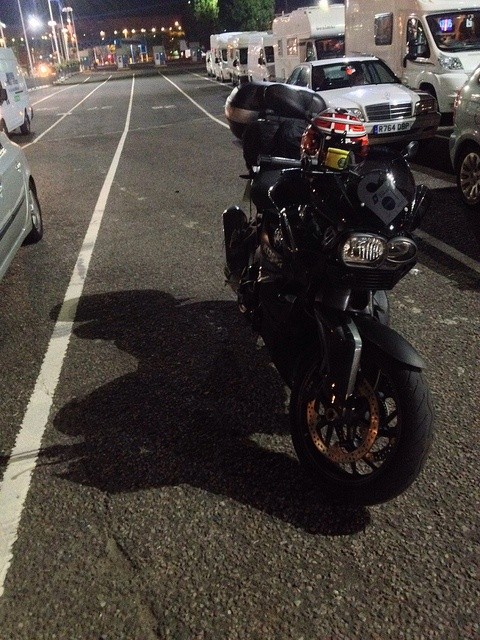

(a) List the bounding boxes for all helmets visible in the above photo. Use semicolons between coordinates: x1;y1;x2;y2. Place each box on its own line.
300;106;369;172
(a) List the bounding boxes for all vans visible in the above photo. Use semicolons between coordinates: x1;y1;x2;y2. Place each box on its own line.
343;1;480;132
227;31;267;89
272;4;345;84
0;46;35;138
247;31;275;83
210;32;243;85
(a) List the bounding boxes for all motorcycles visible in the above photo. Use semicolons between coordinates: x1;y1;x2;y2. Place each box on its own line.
221;79;433;505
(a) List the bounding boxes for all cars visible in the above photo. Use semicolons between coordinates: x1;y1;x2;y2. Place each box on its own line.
206;50;215;77
448;62;480;207
0;129;43;281
283;54;442;156
